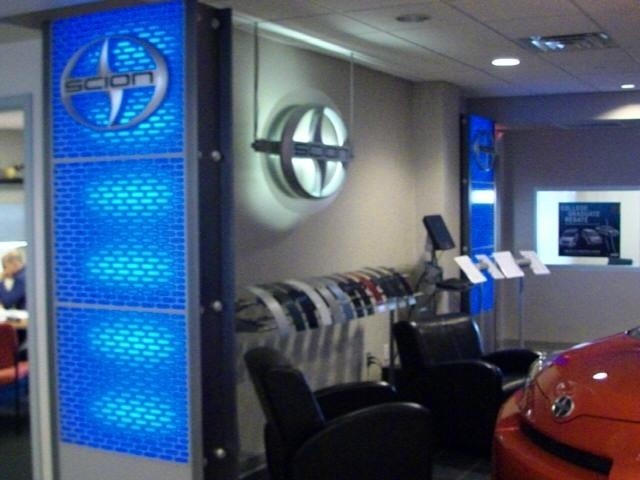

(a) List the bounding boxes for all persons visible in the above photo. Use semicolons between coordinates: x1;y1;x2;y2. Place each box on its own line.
0;251;28;360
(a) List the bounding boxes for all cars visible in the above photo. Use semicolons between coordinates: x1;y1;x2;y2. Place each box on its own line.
560;225;619;249
491;323;639;480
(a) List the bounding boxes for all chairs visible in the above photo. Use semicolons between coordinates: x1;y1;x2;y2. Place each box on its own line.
0;321;29;436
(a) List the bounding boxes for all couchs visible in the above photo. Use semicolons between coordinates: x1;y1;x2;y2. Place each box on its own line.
246;347;432;480
394;313;541;447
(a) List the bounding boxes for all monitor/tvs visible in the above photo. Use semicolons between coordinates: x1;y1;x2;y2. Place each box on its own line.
423;216;454;249
